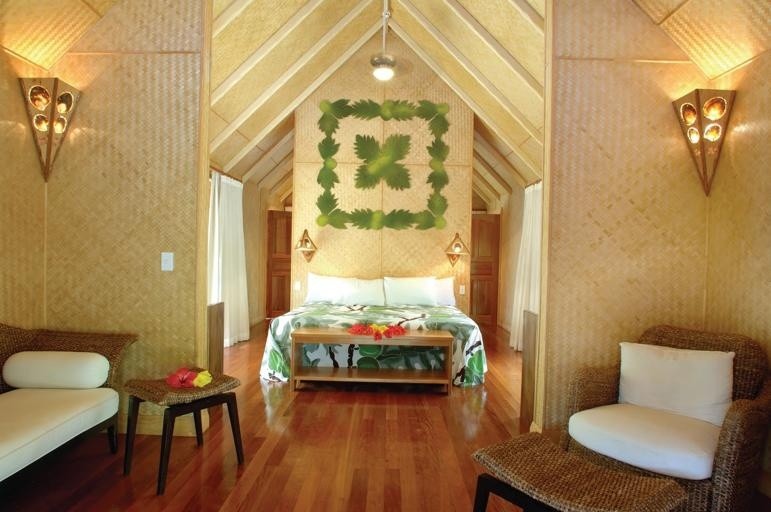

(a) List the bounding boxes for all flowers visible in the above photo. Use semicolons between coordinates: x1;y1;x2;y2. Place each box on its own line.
163;366;195;389
346;323;407;342
189;371;213;389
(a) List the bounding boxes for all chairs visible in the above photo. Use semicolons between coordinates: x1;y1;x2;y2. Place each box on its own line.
553;320;769;512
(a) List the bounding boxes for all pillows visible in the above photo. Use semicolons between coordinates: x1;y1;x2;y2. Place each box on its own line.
0;348;109;390
611;334;736;431
302;271;385;308
380;275;440;307
437;275;456;308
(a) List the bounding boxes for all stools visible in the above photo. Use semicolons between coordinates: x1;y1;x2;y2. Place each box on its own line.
121;369;245;497
464;428;689;512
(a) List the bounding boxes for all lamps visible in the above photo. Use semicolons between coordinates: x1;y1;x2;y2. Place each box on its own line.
16;73;86;183
665;88;741;200
368;0;397;82
445;232;471;268
293;227;318;264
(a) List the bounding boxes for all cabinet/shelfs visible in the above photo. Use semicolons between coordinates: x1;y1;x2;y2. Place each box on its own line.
288;326;456;398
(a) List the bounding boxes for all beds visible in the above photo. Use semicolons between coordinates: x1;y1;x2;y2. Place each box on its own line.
256;303;489;389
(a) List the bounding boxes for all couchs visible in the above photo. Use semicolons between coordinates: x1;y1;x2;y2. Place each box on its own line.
0;320;139;490
